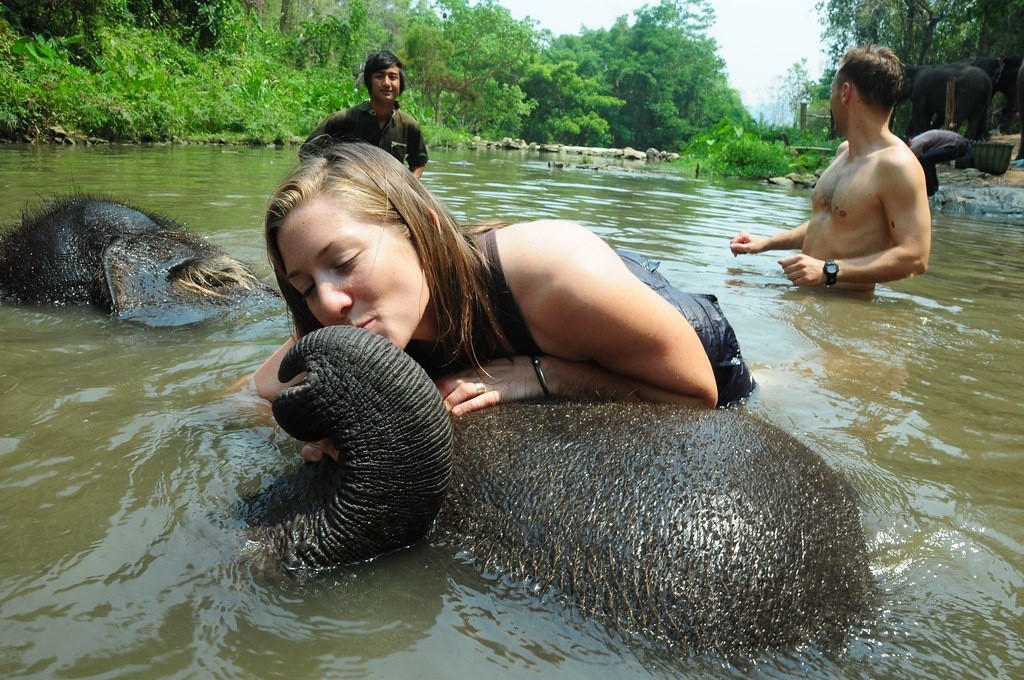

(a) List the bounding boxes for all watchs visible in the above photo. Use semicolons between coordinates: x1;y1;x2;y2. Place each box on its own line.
823;257;839;284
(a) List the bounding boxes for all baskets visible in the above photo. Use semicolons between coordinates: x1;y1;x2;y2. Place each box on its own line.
970;140;1015;175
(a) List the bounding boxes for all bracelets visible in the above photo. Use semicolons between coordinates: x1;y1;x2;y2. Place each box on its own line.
528;351;549;397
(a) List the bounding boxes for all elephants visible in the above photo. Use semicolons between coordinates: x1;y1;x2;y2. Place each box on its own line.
0;182;875;658
899;54;1024;160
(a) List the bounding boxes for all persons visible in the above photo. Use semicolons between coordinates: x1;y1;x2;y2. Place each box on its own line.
251;142;759;469
729;44;931;292
898;129;968;196
299;49;429;183
